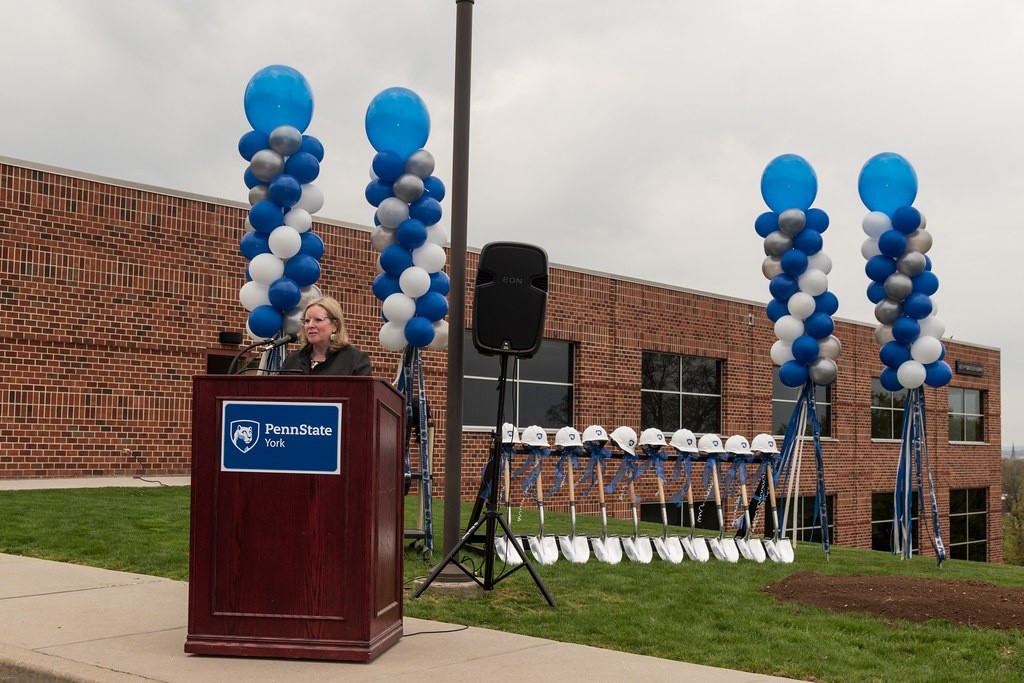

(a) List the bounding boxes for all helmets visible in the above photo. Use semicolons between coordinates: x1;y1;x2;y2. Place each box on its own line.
521;425;550;446
502;422;521;443
724;435;754;454
698;433;726;452
609;426;637;456
751;433;780;453
555;426;583;446
638;428;668;446
582;425;608;443
669;428;698;453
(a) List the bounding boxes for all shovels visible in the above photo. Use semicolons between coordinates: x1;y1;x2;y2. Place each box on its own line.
591;458;623;565
736;464;766;564
493;449;524;565
680;470;710;564
652;476;684;564
527;450;560;565
557;455;590;564
707;463;739;564
763;464;794;564
621;470;653;563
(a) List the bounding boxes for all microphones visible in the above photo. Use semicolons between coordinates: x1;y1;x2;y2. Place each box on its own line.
238;368;303;376
261;333;298;353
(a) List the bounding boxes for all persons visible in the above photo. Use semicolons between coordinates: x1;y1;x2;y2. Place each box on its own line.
278;296;372;376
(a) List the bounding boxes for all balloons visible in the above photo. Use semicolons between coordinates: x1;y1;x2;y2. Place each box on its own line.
857;152;953;392
238;63;325;342
754;153;842;386
364;86;451;351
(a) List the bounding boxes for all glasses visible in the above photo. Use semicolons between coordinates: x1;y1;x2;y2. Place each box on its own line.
300;316;337;326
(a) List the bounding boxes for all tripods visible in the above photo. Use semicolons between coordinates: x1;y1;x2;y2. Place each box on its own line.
409;355;559;609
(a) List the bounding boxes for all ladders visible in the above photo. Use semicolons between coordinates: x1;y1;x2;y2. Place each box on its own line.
400;395;435;562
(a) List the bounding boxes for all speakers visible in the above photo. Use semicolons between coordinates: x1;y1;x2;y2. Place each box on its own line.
472;241;549;360
